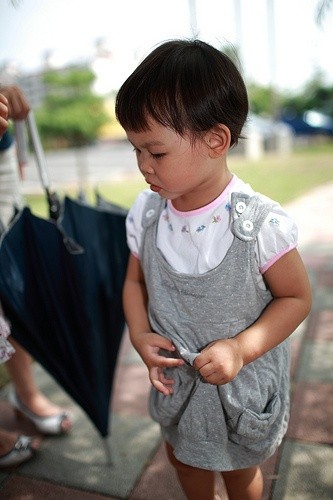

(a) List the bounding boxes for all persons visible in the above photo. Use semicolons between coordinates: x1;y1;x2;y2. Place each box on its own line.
1;73;74;471
112;37;311;498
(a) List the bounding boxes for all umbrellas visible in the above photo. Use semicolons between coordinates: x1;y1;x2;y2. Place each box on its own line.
1;89;146;470
